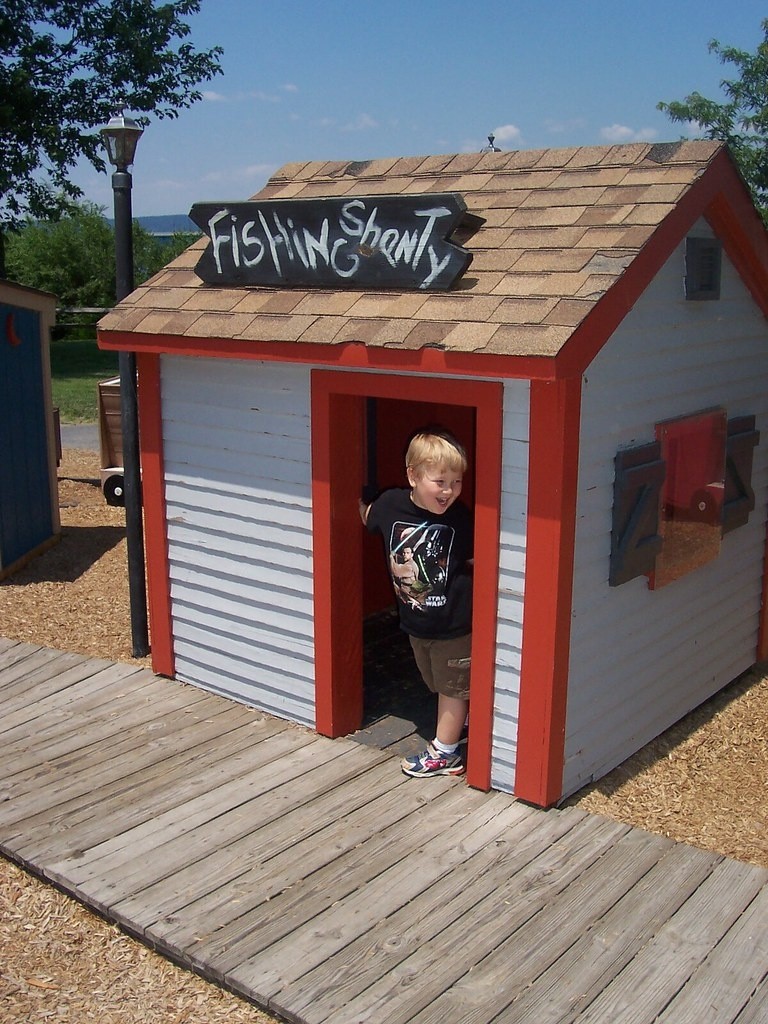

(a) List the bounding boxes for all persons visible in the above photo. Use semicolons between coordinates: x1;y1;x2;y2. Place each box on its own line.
360;430;475;779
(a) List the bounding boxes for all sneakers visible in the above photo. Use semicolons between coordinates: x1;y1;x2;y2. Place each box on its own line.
400;745;466;778
431;723;468;745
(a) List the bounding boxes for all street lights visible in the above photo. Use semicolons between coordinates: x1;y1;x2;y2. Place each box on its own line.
101;97;153;659
